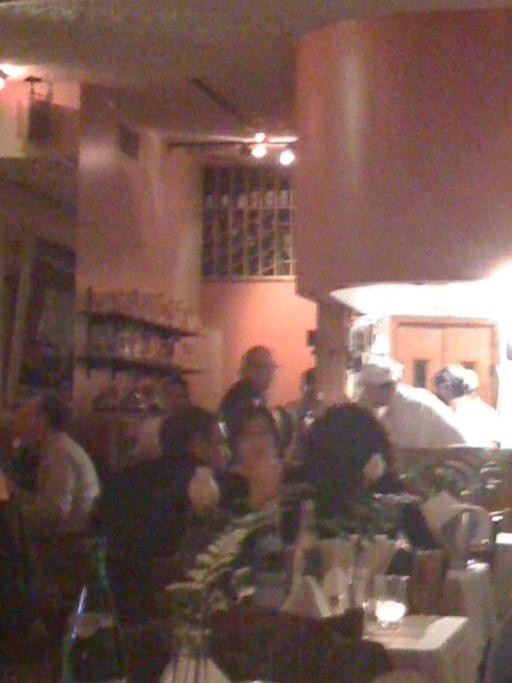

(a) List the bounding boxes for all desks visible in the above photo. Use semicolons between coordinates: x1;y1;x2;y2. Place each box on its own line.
159;503;512;683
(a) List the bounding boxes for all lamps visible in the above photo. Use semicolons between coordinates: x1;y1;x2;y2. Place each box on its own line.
170;141;297;166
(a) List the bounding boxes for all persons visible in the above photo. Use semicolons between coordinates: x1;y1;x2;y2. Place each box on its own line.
2;342;510;681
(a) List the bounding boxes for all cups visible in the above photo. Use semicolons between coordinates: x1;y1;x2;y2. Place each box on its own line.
372;574;409;629
455;511;489;556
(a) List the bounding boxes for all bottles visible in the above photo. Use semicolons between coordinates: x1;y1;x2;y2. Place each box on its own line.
61;534;126;683
292;500;325;583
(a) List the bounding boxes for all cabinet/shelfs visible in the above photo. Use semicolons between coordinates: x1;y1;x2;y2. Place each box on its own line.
80;309;204;378
